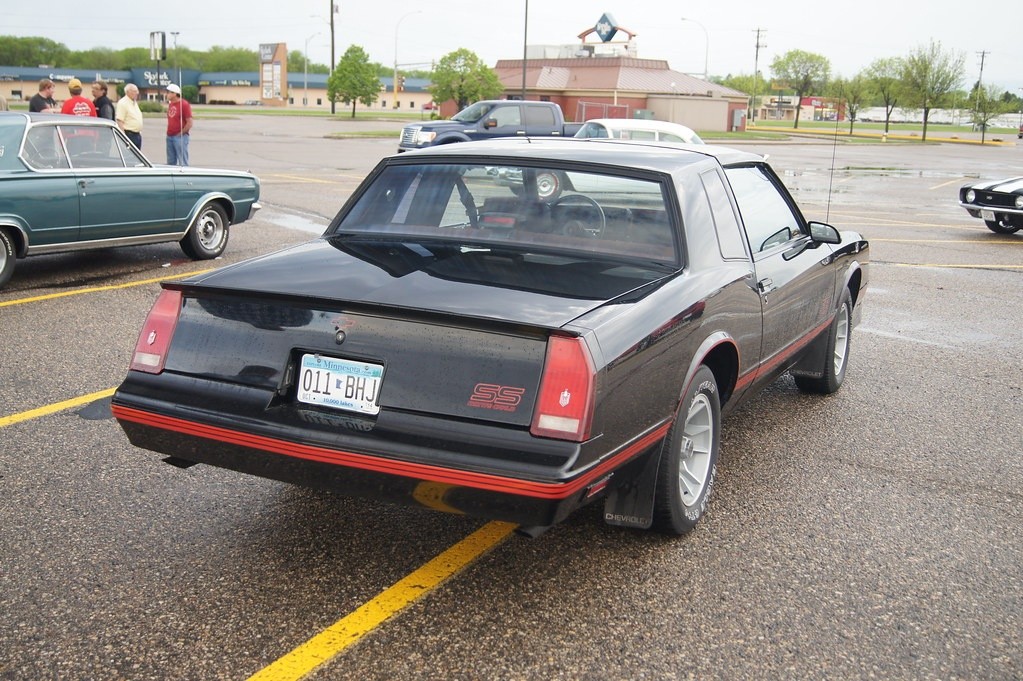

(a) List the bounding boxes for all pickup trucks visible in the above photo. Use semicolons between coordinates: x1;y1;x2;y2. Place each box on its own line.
395;100;628;179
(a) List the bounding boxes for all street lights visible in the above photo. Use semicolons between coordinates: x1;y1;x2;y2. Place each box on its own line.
309;0;336;114
305;32;322;104
393;10;423;109
680;17;708;81
170;31;179;84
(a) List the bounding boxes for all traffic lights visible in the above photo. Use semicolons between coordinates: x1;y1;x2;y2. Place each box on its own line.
432;59;435;70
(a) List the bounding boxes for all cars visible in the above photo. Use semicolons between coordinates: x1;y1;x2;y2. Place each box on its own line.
0;112;262;290
110;139;868;537
959;176;1023;233
486;119;771;211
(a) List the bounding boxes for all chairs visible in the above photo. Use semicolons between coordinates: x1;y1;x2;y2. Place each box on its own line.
474;198;553;233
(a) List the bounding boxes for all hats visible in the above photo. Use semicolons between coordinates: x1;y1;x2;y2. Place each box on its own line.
68;78;82;90
166;83;181;94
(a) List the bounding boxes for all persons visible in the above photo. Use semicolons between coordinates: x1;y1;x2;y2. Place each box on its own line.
89;82;115;157
29;79;61;158
115;83;143;151
166;83;192;166
60;78;99;153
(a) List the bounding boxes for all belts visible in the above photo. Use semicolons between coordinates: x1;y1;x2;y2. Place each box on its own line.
130;131;141;134
